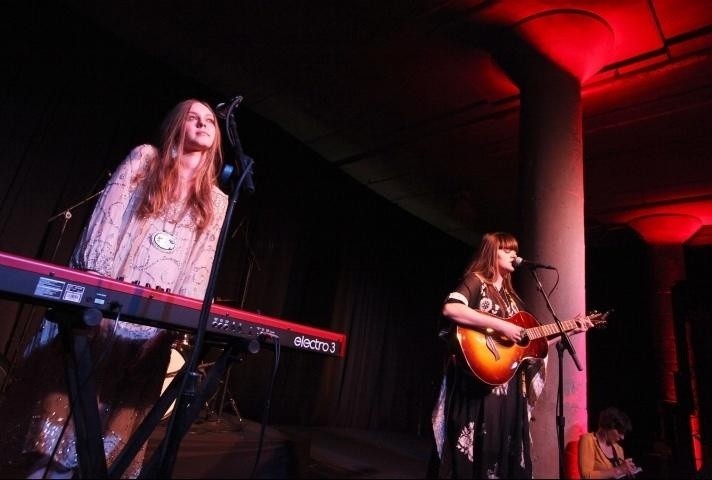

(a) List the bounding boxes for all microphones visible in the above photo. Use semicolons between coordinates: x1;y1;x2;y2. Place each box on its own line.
215;95;244;120
514;256;555;270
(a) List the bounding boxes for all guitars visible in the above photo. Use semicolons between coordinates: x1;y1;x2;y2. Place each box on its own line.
456;310;609;387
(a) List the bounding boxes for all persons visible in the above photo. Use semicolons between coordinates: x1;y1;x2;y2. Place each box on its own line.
417;231;597;478
16;99;230;478
578;407;643;479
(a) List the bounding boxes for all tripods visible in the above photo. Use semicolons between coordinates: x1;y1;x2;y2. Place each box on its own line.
205;364;248;440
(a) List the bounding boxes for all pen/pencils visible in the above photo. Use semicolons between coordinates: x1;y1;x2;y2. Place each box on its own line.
618;457;633;467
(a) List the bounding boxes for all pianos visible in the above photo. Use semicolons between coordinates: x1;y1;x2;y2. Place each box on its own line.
1;254;346;361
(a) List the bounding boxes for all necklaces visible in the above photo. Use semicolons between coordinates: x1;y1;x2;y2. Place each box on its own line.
152;213;178;253
